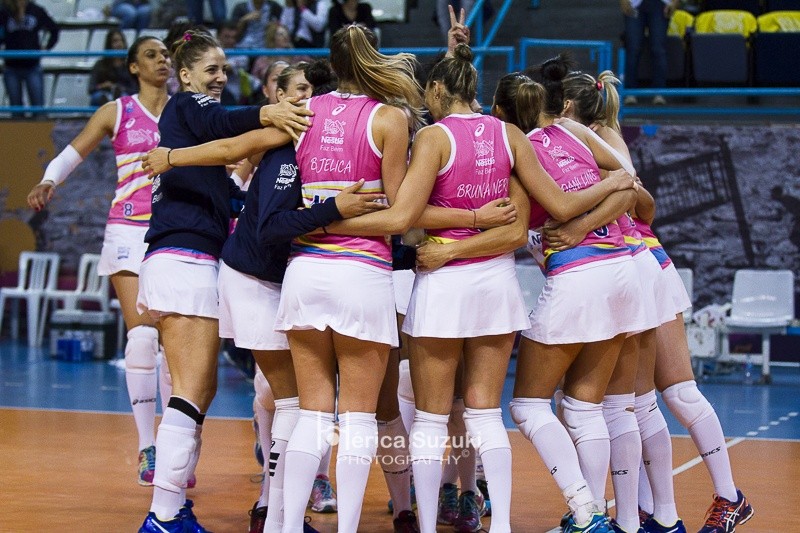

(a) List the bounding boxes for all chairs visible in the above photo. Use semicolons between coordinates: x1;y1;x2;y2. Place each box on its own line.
621;10;800;102
0;252;60;348
0;0;406;118
36;255;109;346
724;269;795;381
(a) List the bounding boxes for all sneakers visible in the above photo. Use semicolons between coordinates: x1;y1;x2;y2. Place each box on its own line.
639;511;654;527
437;483;459;525
136;445;156;486
545;511;615;533
303;516;320;533
476;479;491;517
605;513;615;523
186;474;196;488
387;473;418;513
643;516;687;533
393;510;420;533
248;501;268;533
453;490;482;533
475;487;488;518
610;518;650;533
138;498;216;533
309;474;338;513
697;489;754;533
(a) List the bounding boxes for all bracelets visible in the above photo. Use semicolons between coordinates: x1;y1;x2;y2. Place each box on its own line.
322;226;328;233
168;149;173;167
467;208;478;228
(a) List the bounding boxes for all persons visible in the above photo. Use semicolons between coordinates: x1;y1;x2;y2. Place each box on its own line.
0;0;756;533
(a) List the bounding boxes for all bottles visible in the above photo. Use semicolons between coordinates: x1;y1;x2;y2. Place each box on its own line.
56;328;94;361
742;364;753;385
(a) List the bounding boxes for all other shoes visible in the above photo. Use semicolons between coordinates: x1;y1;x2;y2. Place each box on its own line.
253;414;264;467
624;95;636;104
653;95;665;105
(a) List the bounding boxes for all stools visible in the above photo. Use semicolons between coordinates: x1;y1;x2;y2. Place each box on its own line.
48;309;115;359
